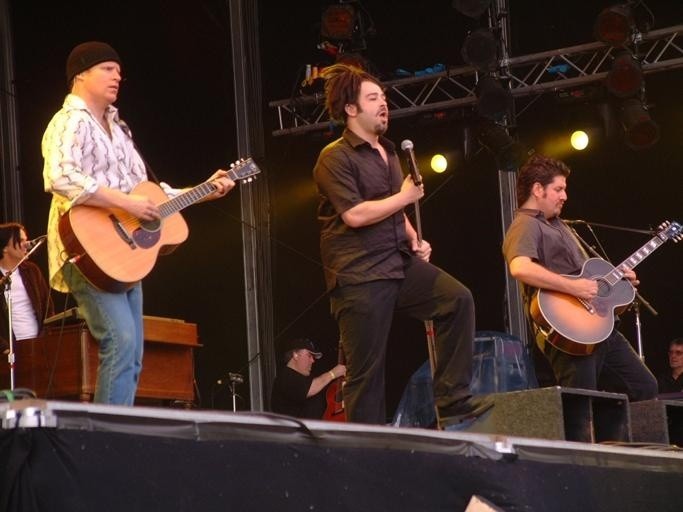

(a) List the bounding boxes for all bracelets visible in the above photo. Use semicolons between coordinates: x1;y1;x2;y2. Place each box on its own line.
328;371;335;379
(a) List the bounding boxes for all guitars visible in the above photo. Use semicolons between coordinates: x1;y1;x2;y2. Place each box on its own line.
323;333;347;422
58;158;261;293
530;221;683;355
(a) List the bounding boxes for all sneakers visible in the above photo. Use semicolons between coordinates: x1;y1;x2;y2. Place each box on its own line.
436;395;494;427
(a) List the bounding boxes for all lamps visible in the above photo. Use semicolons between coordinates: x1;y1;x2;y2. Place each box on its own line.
570;1;663;154
292;1;358;151
453;1;513;176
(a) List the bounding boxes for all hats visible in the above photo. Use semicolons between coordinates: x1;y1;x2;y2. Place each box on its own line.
67;42;119;81
279;334;323;360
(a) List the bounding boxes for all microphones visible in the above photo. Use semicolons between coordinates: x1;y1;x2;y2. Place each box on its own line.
24;235;47;251
399;138;423;186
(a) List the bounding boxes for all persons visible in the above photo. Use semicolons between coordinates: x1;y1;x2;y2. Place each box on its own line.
500;155;658;440
656;337;682;393
1;223;54;398
39;41;236;405
313;63;496;430
270;339;347;418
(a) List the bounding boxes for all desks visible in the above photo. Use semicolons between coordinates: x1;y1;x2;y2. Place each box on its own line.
0;316;203;410
